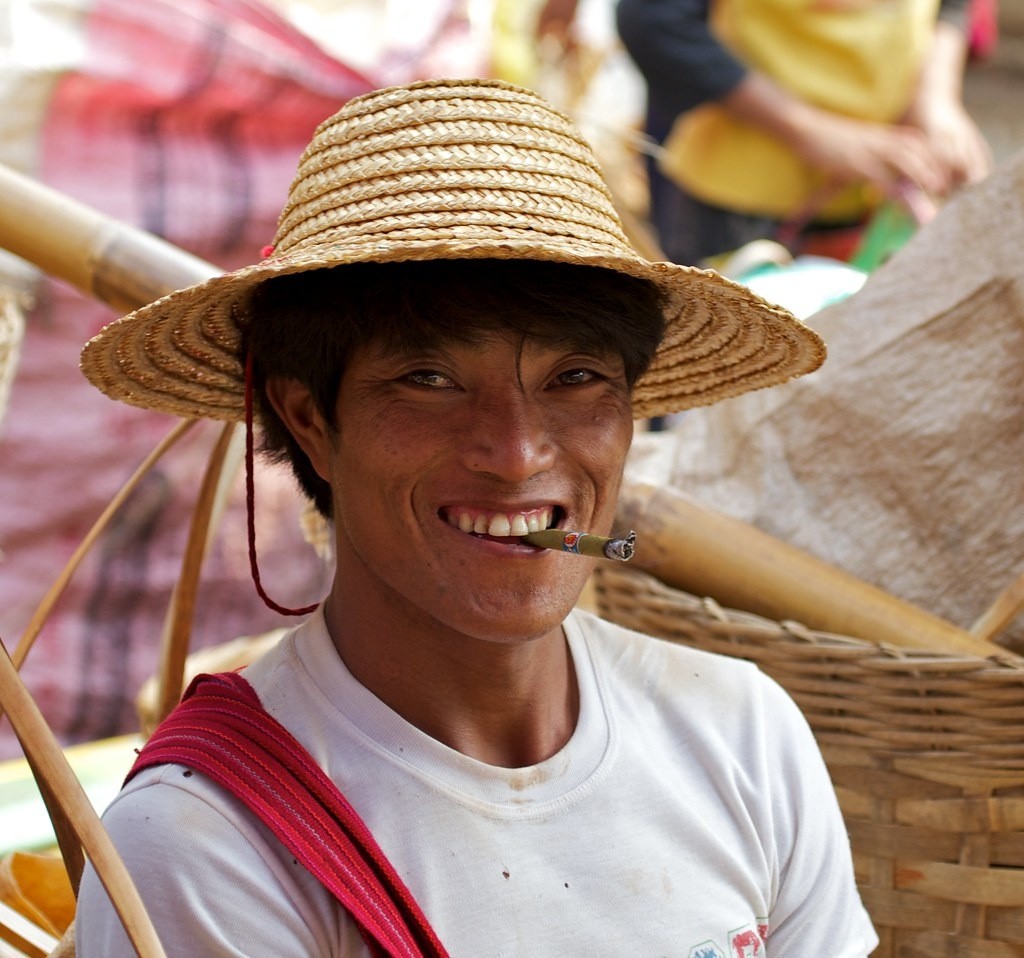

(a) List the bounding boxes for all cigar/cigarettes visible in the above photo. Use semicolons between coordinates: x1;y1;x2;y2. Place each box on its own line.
529;525;638;564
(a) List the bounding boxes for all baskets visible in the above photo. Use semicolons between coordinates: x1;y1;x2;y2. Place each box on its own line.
586;564;1024;958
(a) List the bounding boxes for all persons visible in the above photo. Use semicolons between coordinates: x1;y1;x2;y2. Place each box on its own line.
0;0;1024;958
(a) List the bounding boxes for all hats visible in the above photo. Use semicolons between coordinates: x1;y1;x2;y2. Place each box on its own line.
80;76;830;419
